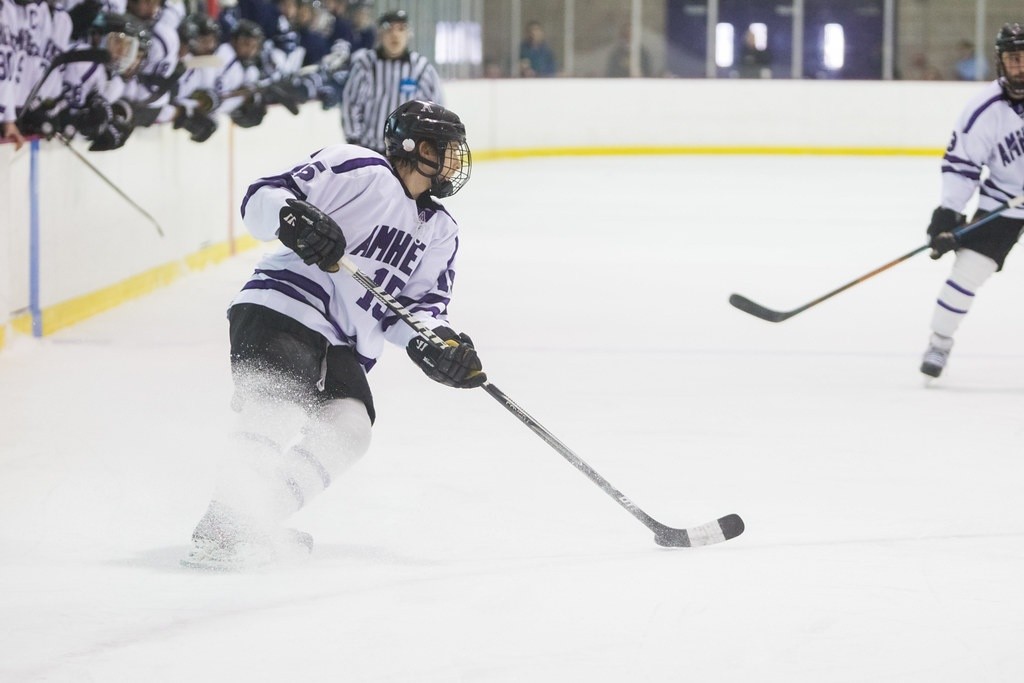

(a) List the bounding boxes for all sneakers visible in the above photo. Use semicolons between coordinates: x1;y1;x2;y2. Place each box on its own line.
919;342;950;388
181;500;312;573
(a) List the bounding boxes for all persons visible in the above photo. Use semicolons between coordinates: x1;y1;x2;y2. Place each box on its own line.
920;23;1024;381
607;24;650;78
484;23;555;77
739;31;772;78
342;9;443;153
183;99;488;568
914;39;988;81
0;0;375;151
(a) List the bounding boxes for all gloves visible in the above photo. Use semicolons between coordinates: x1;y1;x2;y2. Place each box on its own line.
926;206;967;260
73;86;343;152
407;325;487;389
276;197;346;273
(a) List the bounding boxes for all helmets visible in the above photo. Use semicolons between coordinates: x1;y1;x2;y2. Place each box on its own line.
379;10;409;28
91;0;376;86
384;99;472;199
995;23;1024;99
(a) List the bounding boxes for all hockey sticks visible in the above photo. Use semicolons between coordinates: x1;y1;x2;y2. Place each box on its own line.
142;55;222;104
338;254;745;549
729;194;1024;323
53;131;165;239
